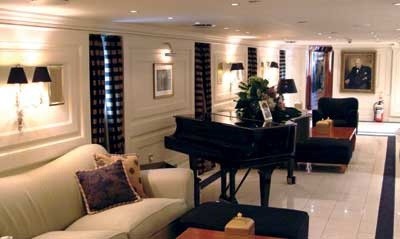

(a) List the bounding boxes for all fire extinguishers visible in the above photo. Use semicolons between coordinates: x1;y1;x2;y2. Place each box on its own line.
373;100;384;122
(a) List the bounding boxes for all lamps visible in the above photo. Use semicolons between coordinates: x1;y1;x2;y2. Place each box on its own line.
269;61;279;69
31;66;53;105
230;63;245;80
279;79;298;94
6;66;29;111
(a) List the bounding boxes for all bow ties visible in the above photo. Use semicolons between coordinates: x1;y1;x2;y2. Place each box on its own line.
356;67;359;70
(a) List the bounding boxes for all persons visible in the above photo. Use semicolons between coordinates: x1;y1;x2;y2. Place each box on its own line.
345;58;371;89
262;103;271;118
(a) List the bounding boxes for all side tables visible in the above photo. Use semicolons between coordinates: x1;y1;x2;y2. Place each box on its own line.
139;160;201;206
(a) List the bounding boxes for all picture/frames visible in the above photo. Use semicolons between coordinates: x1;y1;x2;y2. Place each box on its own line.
153;62;174;100
257;99;273;121
340;49;377;94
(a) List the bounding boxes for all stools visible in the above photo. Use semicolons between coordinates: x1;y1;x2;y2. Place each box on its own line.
172;199;309;239
295;137;353;174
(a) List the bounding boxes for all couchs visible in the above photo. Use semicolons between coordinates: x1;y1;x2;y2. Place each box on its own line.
0;144;195;239
312;96;359;133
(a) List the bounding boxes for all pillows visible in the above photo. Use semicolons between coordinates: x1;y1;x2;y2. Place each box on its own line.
90;150;149;198
73;158;141;215
